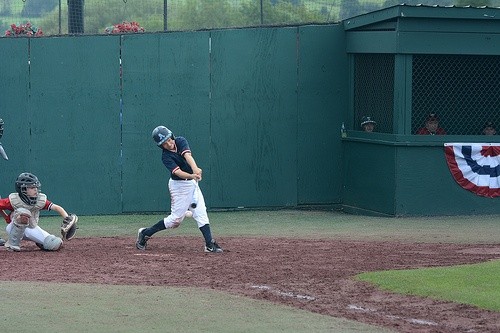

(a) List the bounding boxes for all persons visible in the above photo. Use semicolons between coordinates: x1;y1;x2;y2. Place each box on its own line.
360;113;377;132
0;172;79;251
136;125;222;253
416;113;445;135
481;121;498;135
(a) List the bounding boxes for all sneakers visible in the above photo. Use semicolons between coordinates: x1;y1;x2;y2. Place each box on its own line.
205;241;224;253
135;227;152;250
4;241;21;252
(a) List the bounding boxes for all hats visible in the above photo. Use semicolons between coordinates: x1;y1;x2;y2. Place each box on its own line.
483;121;496;129
427;112;440;120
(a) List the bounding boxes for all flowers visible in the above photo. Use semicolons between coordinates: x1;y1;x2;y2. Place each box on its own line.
104;19;146;34
3;22;44;38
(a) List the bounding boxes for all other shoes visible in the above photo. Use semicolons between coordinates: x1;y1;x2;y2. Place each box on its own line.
0;238;6;245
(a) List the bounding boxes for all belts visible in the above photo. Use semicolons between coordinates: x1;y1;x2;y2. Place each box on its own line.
175;177;192;180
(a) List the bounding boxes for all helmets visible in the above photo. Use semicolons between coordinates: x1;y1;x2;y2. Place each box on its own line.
15;172;42;205
360;115;377;128
152;125;172;146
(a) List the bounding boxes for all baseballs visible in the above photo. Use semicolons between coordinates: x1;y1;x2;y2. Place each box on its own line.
185;211;193;218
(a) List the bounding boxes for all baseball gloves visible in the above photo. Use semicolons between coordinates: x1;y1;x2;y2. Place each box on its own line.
60;213;79;241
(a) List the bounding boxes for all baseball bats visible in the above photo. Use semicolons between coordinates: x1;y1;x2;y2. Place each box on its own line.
191;177;199;208
